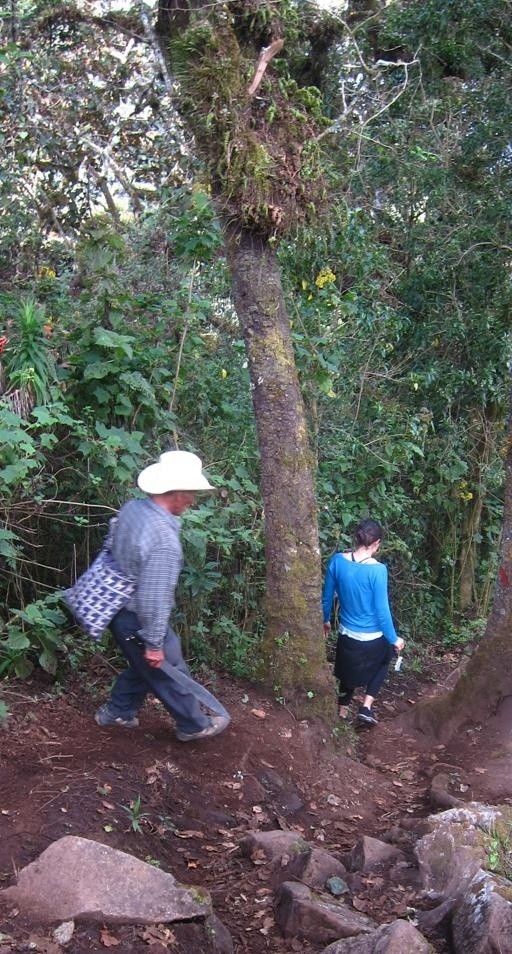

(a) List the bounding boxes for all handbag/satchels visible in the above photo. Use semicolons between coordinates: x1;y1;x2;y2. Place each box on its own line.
55;546;139;641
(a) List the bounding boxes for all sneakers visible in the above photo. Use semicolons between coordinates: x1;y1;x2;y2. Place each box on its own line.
357;706;380;727
93;705;141;731
174;711;232;745
337;716;353;727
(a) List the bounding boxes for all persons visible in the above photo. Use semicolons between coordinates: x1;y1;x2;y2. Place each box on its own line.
321;518;405;724
94;450;230;741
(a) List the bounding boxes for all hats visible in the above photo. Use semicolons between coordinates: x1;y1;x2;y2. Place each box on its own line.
136;450;220;497
